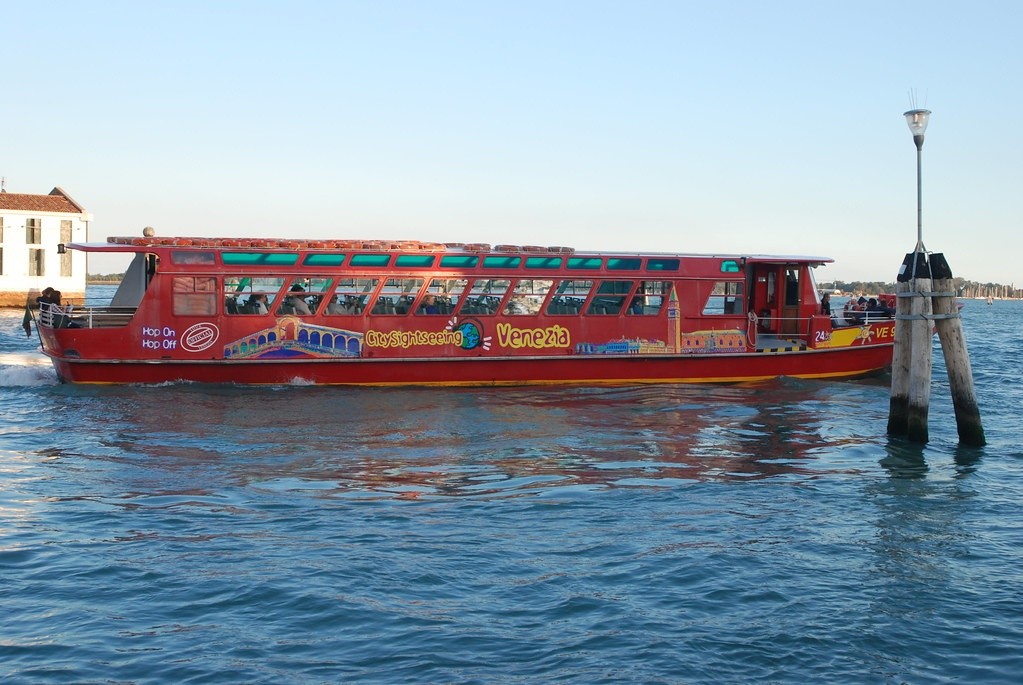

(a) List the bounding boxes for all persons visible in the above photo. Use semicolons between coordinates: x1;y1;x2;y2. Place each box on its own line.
842;294;896;325
822;294;838;328
287;283;313;316
36;287;73;327
425;295;441;314
634;296;644;315
249;290;267;315
329;295;359;316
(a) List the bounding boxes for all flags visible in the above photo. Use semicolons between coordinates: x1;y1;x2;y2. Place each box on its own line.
22;301;32;339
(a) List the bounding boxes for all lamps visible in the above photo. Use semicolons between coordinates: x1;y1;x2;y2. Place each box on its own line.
57;244;69;255
740;257;746;267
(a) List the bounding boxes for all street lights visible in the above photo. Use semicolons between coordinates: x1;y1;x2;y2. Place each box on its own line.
902;104;930;249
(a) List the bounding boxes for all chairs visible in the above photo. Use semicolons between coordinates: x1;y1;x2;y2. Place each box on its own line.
225;295;634;317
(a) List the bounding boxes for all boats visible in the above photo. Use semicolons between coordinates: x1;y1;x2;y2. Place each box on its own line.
19;227;967;386
